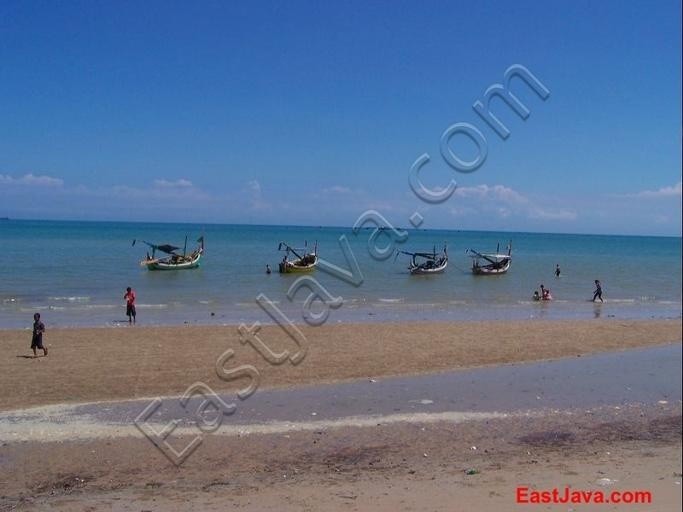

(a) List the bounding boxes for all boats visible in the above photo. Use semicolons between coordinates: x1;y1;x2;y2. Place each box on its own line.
460;238;513;276
393;245;450;274
276;238;319;273
132;234;206;271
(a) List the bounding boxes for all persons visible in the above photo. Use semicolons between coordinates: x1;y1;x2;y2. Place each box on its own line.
265;263;271;273
540;284;545;299
542;289;551;300
123;287;136;322
554;264;560;276
592;280;603;303
30;311;47;358
532;291;539;300
